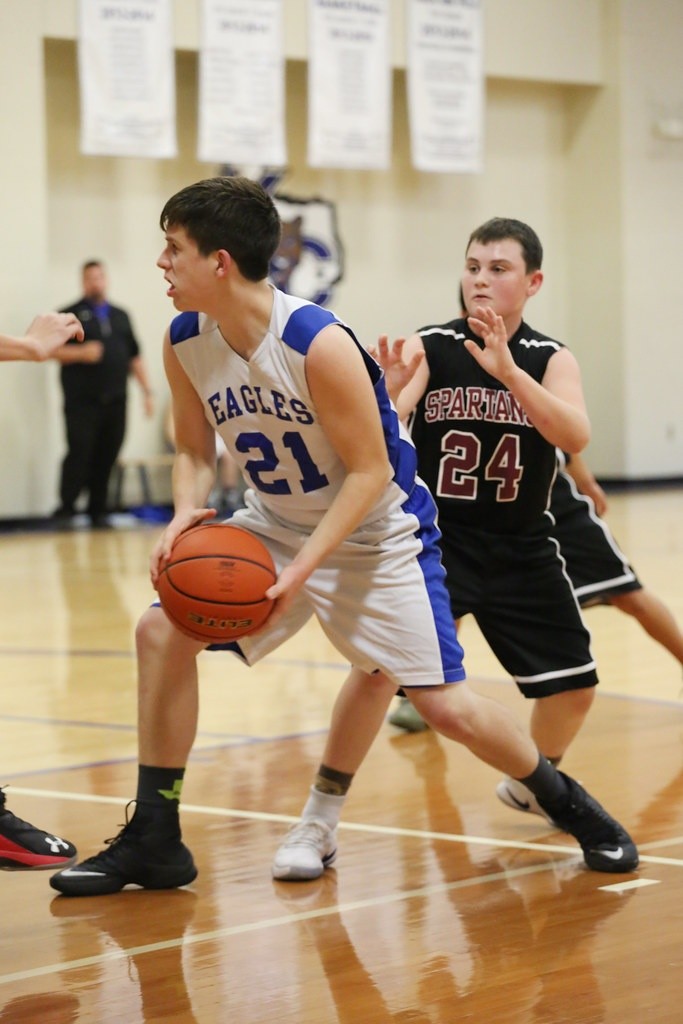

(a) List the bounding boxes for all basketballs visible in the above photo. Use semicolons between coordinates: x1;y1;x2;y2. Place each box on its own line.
154;522;280;645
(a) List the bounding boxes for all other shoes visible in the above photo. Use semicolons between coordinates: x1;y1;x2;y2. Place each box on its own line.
53;506;114;530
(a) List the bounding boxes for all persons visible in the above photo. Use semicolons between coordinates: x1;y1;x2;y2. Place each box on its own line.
386;277;683;733
0;311;84;872
47;177;640;897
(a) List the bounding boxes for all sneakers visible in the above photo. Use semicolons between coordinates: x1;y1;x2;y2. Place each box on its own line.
497;776;558;829
271;784;346;880
0;788;78;871
389;696;430;731
50;803;198;897
535;770;638;873
